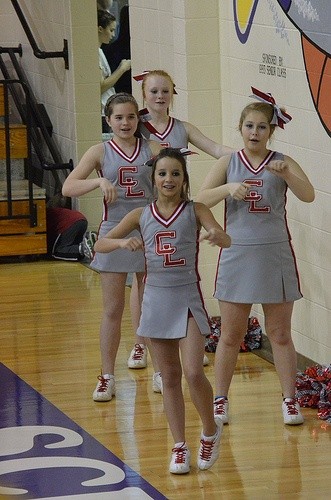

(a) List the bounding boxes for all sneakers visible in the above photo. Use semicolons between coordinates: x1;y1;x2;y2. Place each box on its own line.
153;372;163;396
169;442;192;474
203;354;209;366
198;417;223;470
93;374;116;401
213;398;230;424
81;236;95;260
89;231;98;247
282;398;303;425
128;344;148;368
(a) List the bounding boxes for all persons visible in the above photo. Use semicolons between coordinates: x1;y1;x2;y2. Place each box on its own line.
111;67;240;370
93;147;233;473
32;202;99;262
61;92;182;401
199;88;316;426
79;10;135;179
